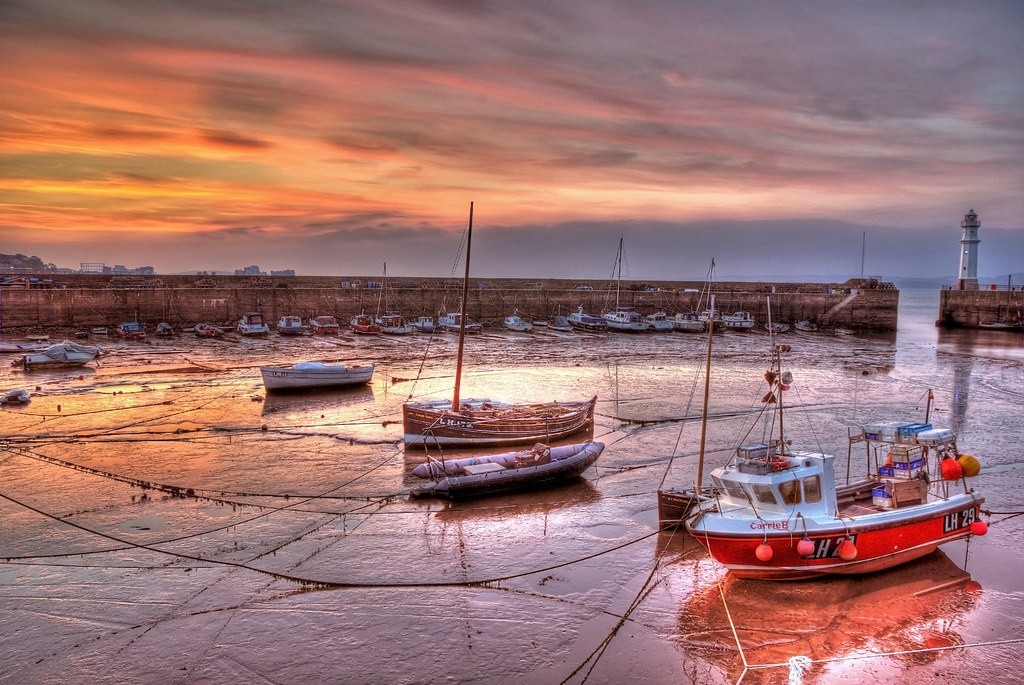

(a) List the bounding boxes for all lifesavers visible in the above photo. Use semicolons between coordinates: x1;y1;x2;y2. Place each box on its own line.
758;455;789;471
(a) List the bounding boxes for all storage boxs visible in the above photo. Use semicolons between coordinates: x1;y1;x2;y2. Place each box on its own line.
872;445;928;510
865;420;954;444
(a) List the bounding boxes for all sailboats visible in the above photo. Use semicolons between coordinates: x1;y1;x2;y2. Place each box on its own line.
374;261;410;324
978;274;1024;330
657;295;887;533
600;237;650;330
693;257;727;332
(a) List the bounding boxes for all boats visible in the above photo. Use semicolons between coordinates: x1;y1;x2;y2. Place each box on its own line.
260;362;375;393
414;442;605;498
684;296;986;582
378;315;412;335
194;323;225;338
155;322;174;338
677;546;983;685
350;309;380;334
23;340;98;370
548;304;574;331
277;316;307;335
765;323;790;333
794;320;818;331
308;315;339;335
438;312;482;332
641;311;673;332
91;327;108;334
237;312;269;336
533;320;549;325
504;309;534;332
835;329;854;335
722;311;756;330
75;332;89;339
0;389;30;404
402;200;598;449
116;322;146;340
0;342;52;353
409;317;447;333
567;303;608;332
667;309;704;332
26;335;50;340
